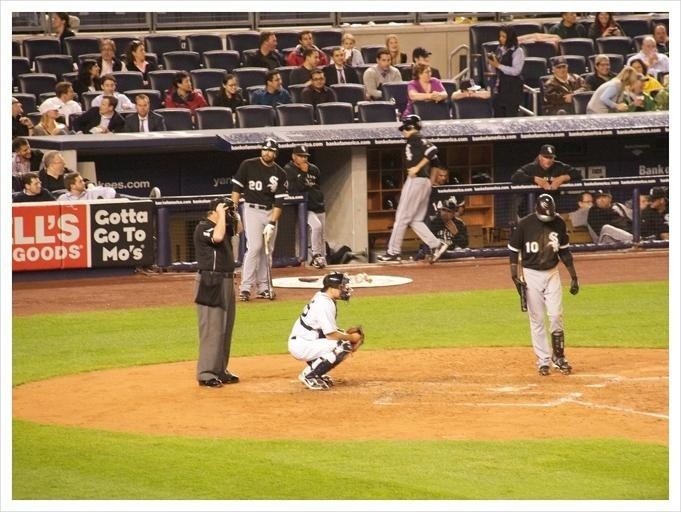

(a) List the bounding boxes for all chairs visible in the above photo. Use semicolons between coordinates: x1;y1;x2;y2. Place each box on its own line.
12;18;668;204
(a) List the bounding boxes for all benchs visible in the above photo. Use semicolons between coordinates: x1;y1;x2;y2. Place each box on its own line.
559;212;594;244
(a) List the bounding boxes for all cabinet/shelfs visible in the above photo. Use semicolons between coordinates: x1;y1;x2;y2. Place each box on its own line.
367;141;495;249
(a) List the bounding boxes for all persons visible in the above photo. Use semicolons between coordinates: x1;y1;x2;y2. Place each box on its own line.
507;193;579;375
231;138;327;300
12;12;669;138
288;272;360;390
12;137;117;203
377;114;668;264
195;195;244;386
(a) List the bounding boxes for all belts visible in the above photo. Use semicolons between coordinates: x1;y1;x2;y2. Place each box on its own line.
196;269;235;278
250;205;266;210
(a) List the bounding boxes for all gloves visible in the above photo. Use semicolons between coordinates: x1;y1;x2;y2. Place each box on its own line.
262;223;275;242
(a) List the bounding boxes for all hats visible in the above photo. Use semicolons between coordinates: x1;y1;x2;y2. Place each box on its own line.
38;99;61;115
399;115;421;129
541;145;556;157
413;47;430;57
293;146;310;156
647;187;665;199
550;57;566;68
592;187;609;197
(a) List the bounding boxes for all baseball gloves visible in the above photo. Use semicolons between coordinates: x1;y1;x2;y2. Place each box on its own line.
348;327;365;352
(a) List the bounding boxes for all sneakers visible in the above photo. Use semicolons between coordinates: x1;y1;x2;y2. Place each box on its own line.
430;243;448;264
198;378;221;386
256;291;271;300
377;253;402;263
221;371;236;383
311;254;324;268
240;291;249;301
299;374;331;391
539;367;549;375
554;362;572;369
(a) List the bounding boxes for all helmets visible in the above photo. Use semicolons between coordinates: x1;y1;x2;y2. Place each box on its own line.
321;275;351;300
263;139;278;152
211;197;239;237
537;194;554;222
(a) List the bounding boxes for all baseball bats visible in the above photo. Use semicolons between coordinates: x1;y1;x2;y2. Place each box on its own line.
518;257;527;312
264;234;275;300
